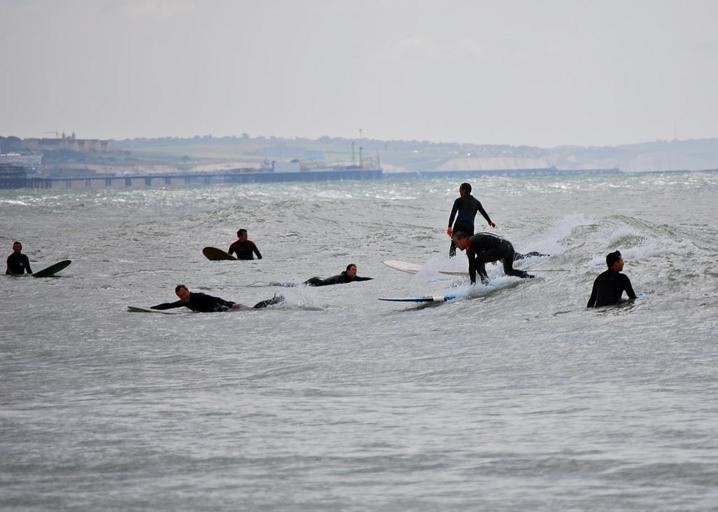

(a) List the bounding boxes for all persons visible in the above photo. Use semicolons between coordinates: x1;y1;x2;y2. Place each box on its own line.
303;264;372;287
586;250;636;308
228;229;262;260
451;231;533;286
150;285;283;313
446;183;494;258
5;241;32;276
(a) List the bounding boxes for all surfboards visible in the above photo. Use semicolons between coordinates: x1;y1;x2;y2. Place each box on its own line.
128;306;193;315
31;260;71;276
377;276;528;302
202;247;234;259
383;259;493;274
